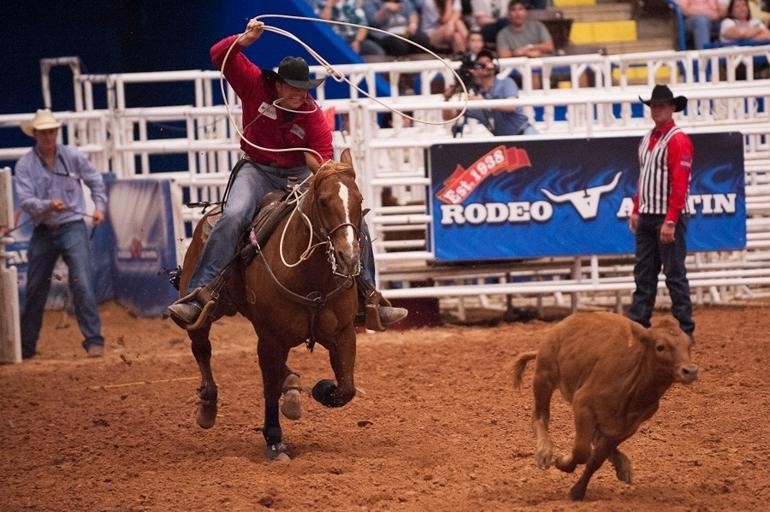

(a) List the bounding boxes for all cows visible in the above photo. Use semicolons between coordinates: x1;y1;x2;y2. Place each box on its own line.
506;310;699;503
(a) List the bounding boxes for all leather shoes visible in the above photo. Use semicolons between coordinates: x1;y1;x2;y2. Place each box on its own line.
84;341;106;358
168;295;213;336
355;302;411;328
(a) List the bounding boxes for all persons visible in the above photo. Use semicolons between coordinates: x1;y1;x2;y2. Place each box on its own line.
440;48;538;136
464;28;485;65
624;82;698;336
166;17;409;328
13;108;109;358
312;1;387;54
719;1;770;42
421;1;469;50
471;0;513;37
495;1;558;88
675;1;726;51
362;0;433;56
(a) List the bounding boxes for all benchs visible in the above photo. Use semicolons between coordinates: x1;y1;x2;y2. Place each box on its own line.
353;0;610;128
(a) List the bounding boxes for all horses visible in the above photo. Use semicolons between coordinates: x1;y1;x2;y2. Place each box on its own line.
178;147;366;464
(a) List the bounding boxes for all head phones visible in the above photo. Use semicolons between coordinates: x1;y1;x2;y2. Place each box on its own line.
491;56;501;73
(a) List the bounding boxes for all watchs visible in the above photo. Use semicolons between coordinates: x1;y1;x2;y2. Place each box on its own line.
664;220;676;227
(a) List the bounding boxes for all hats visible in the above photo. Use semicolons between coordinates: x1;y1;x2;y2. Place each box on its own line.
469;49;494;63
638;84;688;113
19;109;69;139
260;53;326;91
